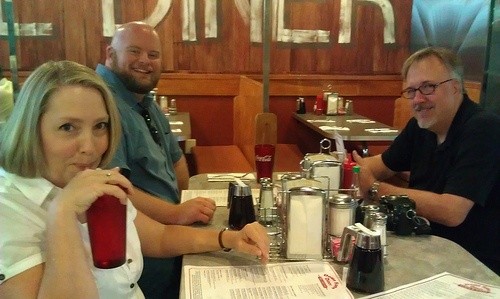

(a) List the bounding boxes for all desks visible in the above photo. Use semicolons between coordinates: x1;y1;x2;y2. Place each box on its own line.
178;175;500;299
295;112;399;142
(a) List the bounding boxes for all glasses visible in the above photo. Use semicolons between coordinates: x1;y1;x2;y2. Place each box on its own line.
400;78;452;99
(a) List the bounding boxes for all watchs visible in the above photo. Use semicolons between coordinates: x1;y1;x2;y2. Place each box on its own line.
368;181;380;200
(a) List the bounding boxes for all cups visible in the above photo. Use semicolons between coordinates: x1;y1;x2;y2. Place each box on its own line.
86;184;127;269
255;143;276;183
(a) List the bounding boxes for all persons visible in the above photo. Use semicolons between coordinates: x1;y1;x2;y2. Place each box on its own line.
0;61;270;299
95;21;217;299
347;48;500;273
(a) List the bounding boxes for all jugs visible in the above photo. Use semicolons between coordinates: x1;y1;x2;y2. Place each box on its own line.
341;222;385;295
296;97;306;114
226;179;256;232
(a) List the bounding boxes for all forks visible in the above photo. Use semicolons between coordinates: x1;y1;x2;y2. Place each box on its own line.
213;171;251;178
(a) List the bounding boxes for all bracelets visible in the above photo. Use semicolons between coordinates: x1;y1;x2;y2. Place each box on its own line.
218;227;231;251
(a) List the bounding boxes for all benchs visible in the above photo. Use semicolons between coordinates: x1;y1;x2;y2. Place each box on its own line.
191;76;303;174
367;98;414;182
166;111;192;141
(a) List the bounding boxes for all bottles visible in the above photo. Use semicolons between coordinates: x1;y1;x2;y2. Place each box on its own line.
258;176;275;224
149;87;176;114
327;149;389;260
338;97;353;115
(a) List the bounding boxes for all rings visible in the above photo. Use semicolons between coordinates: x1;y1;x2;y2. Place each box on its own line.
107;173;111;176
